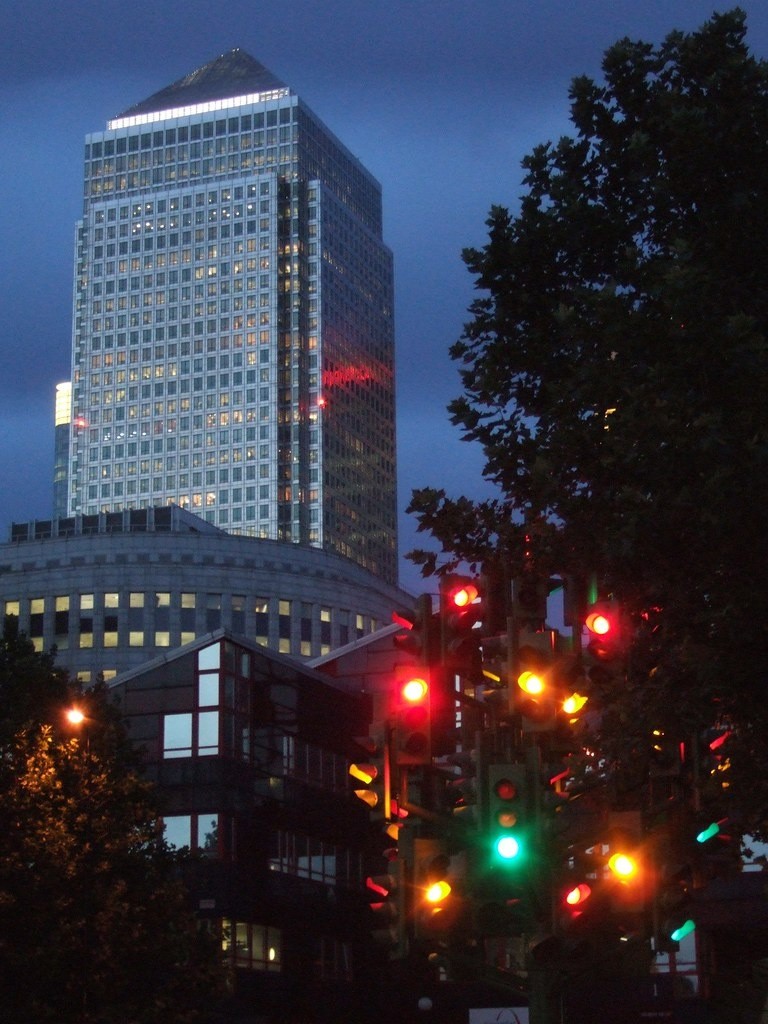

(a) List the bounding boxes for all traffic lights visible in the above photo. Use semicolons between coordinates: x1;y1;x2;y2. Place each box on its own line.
347;742;393;822
538;737;577;818
445;730;483;830
551;874;593;941
488;762;530;873
606;809;647;915
413;839;460;932
557;655;602;737
439;571;481;670
393;664;432;764
580;604;619;687
392;593;433;666
515;631;558;735
366;860;409;947
